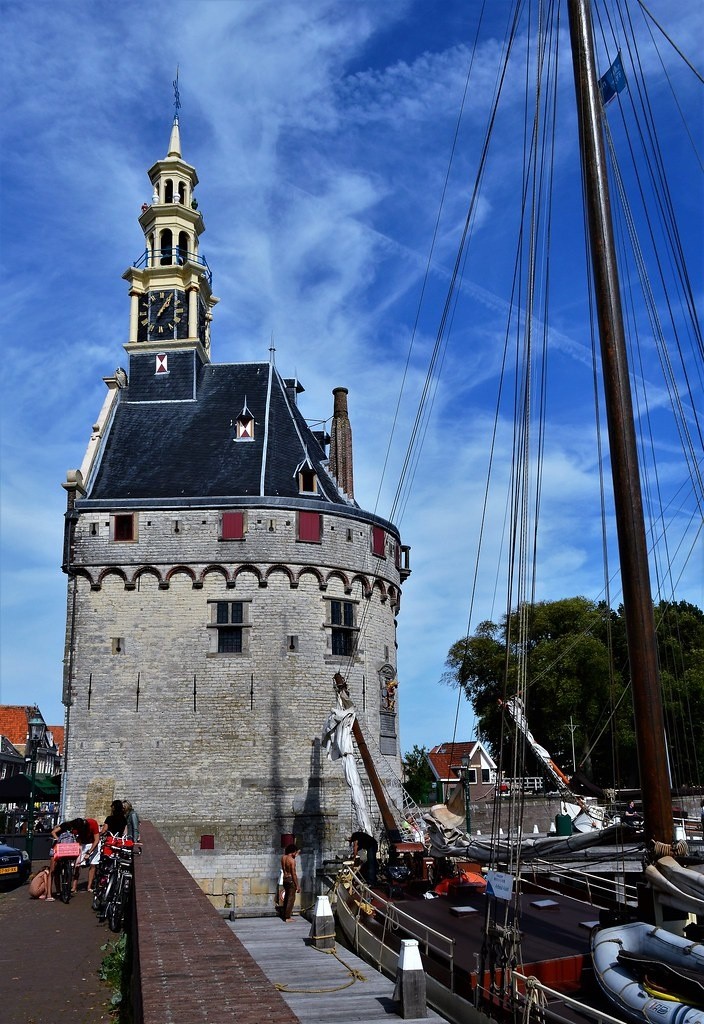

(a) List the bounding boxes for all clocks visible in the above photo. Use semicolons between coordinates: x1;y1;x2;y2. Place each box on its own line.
139;292;184;333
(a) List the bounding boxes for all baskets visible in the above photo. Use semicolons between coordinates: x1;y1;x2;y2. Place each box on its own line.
53;843;80;861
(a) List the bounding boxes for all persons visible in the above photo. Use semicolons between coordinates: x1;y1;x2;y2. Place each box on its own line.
121;800;139;843
281;845;301;922
350;832;378;889
28;866;51;899
100;800;128;838
623;800;644;825
71;818;101;893
7;803;61;833
46;819;76;902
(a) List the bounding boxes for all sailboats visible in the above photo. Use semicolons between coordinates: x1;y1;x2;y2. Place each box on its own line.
317;1;704;1024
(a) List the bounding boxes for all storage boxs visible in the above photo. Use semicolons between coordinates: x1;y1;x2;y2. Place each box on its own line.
53;843;79;860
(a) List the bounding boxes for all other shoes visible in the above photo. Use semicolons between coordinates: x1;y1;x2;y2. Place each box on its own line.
88;889;95;893
46;898;55;902
70;888;77;894
39;895;46;900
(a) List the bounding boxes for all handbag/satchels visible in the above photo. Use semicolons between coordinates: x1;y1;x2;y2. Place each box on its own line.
101;832;133;861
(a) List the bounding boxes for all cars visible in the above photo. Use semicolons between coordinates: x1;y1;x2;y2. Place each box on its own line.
0;840;31;888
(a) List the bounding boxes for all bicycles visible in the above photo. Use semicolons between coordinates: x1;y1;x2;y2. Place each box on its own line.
47;834;81;903
95;831;144;932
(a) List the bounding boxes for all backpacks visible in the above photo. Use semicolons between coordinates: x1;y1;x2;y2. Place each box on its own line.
57;830;77;844
